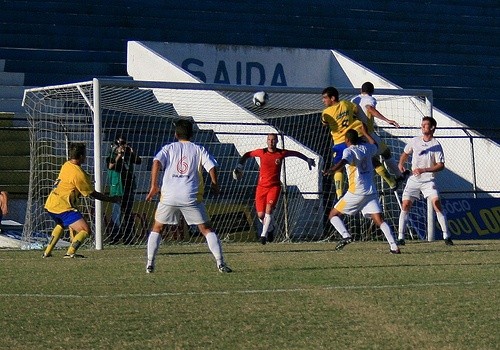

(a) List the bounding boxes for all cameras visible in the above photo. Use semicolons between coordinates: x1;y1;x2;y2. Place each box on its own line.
121;146;132;155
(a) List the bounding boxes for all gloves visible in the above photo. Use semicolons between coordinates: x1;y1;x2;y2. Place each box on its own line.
306;158;316;170
233;164;242;180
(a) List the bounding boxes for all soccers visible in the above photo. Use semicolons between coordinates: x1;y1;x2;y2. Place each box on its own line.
252;91;270;107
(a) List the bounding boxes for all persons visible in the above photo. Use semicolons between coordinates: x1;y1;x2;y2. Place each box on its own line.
233;133;316;245
320;87;403;202
395;116;453;246
105;140;142;245
41;143;122;259
144;119;234;274
321;121;401;255
350;82;410;182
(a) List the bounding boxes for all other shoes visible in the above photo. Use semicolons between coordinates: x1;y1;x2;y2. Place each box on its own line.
392;176;404;190
146;265;154;274
389;248;401;254
260;235;266;245
63;253;84;259
217;264;232;273
394;239;405;245
335;236;353;250
268;225;275;243
444;237;454;245
41;253;55;259
401;169;410;182
